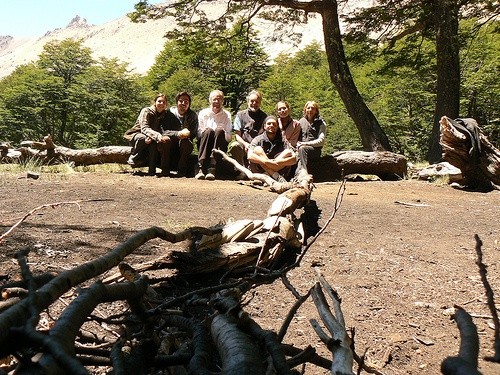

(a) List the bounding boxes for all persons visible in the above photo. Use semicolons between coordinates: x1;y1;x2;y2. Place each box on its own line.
161;92;198;178
194;90;232;180
230;90;268;180
296;101;327;182
274;100;301;151
124;94;170;176
247;116;296;185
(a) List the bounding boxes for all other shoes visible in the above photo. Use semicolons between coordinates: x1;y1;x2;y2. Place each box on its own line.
128;155;290;184
